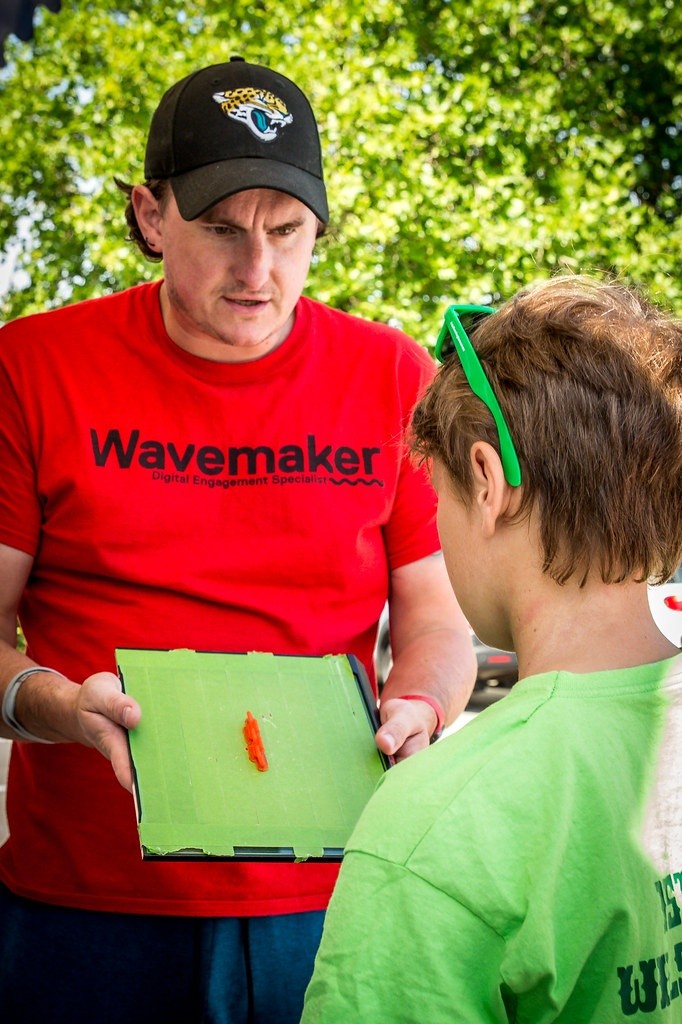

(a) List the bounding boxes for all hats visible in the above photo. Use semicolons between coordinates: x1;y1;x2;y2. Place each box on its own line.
145;55;330;224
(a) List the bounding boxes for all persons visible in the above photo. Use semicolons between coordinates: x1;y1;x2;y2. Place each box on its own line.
301;269;681;1023
0;60;478;1023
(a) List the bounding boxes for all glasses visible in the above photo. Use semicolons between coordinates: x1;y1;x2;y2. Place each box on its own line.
435;304;523;488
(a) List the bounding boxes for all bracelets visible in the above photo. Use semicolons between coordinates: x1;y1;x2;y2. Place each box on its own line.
400;694;444;739
5;667;68;744
